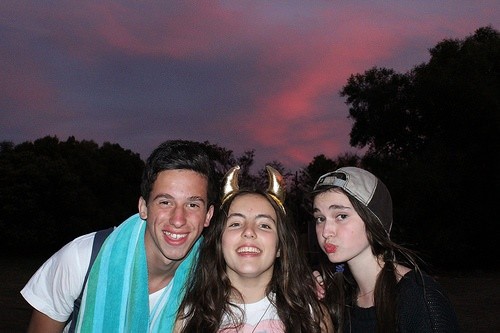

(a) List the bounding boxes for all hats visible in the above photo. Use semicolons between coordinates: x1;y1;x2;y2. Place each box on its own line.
313;166;393;241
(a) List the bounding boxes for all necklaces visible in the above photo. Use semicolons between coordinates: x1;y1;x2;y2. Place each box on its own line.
233;293;273;333
356;290;374;300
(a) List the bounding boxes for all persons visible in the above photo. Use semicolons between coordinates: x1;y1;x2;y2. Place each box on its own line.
309;167;456;333
20;139;228;333
173;166;334;333
